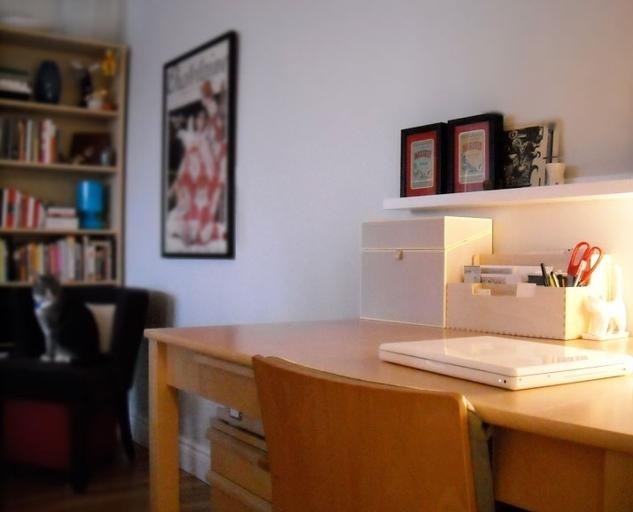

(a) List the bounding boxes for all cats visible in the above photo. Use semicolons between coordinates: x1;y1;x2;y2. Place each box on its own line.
28;269;103;366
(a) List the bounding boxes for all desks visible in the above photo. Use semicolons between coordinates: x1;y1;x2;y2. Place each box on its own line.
144;320;633;511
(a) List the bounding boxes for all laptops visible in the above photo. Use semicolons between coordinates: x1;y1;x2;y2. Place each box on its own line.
379;335;633;391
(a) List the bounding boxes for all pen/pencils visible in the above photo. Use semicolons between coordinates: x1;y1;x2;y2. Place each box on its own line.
540;260;587;288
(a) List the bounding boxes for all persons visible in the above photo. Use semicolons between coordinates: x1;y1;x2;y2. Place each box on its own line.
168;79;228;249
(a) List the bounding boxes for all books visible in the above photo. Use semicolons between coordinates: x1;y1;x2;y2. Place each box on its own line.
2;60;116;284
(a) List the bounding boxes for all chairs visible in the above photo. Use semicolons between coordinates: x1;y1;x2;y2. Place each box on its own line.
250;352;493;512
0;286;149;490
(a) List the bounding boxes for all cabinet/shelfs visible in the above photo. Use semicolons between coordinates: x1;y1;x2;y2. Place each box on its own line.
0;21;127;284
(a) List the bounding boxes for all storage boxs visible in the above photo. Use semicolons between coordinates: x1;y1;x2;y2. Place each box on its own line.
362;218;610;341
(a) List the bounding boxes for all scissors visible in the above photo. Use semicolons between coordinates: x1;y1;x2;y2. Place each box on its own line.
567;240;603;287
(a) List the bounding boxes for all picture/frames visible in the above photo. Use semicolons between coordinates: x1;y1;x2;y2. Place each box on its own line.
160;31;236;259
